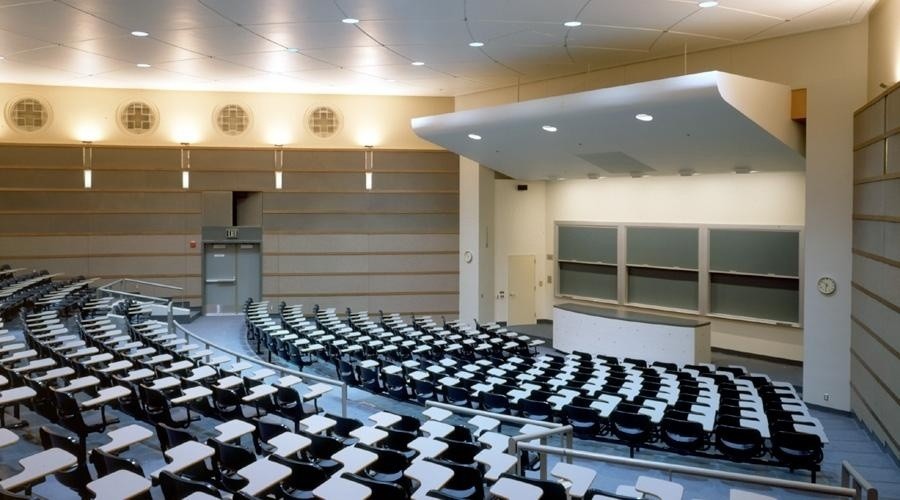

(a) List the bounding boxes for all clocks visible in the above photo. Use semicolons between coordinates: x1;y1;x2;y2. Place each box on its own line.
817;276;836;296
